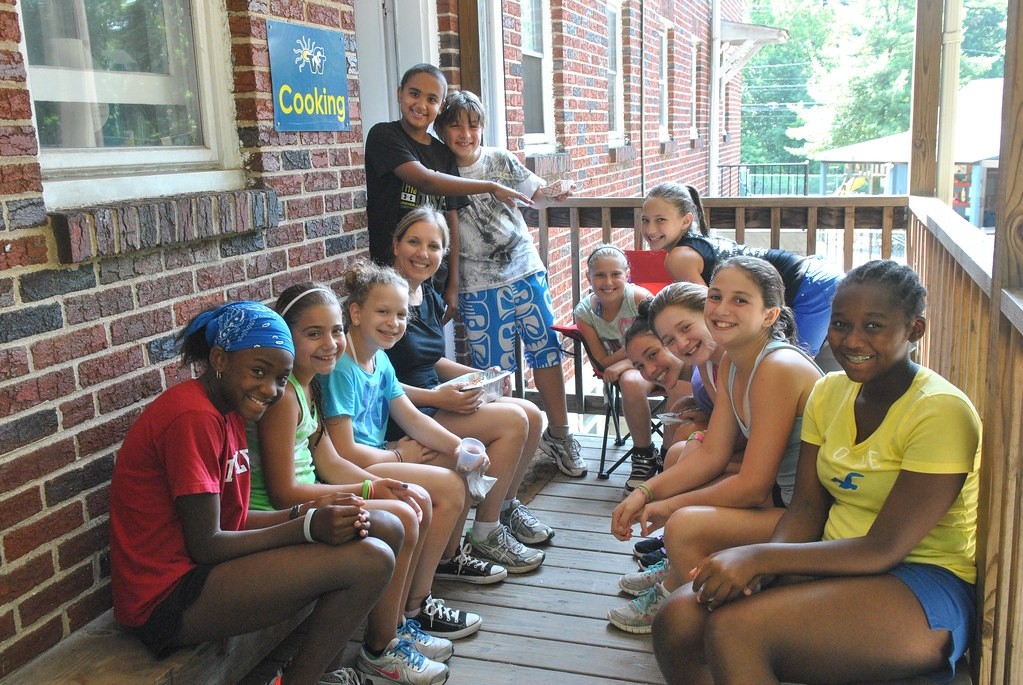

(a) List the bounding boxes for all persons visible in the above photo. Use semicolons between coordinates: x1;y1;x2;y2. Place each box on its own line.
318;259;508;640
108;299;406;684
387;205;555;573
574;180;848;633
651;260;986;685
365;63;583;481
249;284;457;684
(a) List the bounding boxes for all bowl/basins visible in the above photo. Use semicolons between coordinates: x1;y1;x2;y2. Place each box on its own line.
430;369;512;408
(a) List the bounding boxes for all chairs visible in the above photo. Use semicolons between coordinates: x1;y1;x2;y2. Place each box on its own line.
549;247;679;480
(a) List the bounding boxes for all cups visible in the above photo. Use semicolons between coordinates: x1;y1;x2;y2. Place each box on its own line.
456;437;486;478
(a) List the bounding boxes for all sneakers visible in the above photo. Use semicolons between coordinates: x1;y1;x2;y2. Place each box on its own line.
618;558;670;597
624;443;659;495
638;545;666;571
269;667;366;685
607;581;672;634
409;595;484;640
358;638;451;685
396;614;454;663
435;545;508;585
463;524;546;573
539;427;587;476
633;533;665;558
500;500;554;543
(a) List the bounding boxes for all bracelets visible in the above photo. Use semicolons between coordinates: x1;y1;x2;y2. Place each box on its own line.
636;483;652;498
287;503;302;518
687;430;707;443
302;507;319;543
361;479;375;499
391;447;402;461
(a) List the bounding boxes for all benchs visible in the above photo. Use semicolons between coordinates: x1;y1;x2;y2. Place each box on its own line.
1;592;322;685
